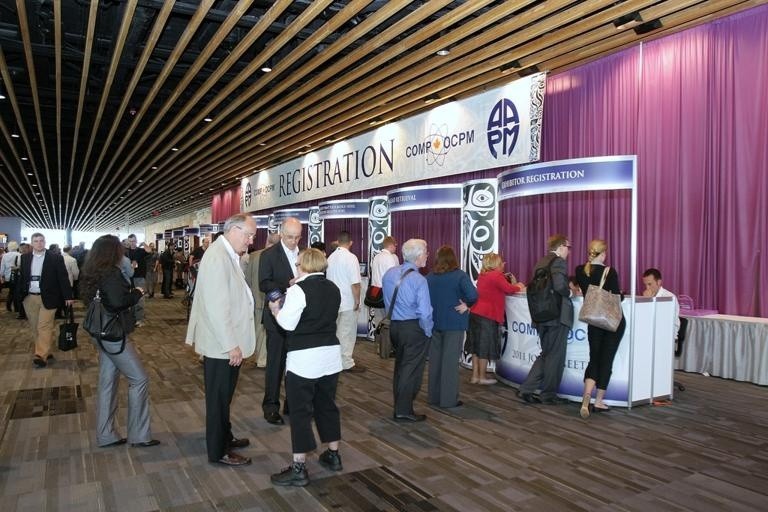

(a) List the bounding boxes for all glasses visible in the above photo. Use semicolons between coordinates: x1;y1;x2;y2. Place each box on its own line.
232;225;257;240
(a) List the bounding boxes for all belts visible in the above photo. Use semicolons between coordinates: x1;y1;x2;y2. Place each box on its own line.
29;293;41;295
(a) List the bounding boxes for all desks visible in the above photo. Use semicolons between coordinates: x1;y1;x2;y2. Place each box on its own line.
675;314;768;385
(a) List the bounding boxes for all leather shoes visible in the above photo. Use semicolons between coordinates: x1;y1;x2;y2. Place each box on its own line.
394;414;426;422
16;311;26;319
542;395;569;404
219;400;343;486
344;364;365;372
457;400;463;406
32;358;45;368
479;378;498;384
579;407;591;419
594;404;611;411
6;303;12;312
516;390;541;403
470;377;479;384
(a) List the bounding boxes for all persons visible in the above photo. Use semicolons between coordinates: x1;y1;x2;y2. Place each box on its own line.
1;236;79;327
163;234;210;322
326;231;366;372
183;212;255;465
515;235;576;404
266;248;344;486
568;274;581;294
20;232;73;366
381;238;436;420
424;244;478;408
464;253;525;386
239;235;280;367
576;240;627;421
642;268;681;351
116;235;161;327
80;235;160;448
371;237;399;358
259;217;304;425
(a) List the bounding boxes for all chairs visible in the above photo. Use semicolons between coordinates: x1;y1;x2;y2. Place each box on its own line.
675;317;687;391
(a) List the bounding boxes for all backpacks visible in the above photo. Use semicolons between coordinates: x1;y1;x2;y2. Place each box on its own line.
526;257;561;322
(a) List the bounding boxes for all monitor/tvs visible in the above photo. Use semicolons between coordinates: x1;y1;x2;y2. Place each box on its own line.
359;261;367;276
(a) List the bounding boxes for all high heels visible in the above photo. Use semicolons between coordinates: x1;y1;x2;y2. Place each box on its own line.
97;438;127;448
131;439;160;447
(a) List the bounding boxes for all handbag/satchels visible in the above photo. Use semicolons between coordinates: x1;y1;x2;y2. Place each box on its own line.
83;288;126;355
579;266;623;333
364;285;385;308
377;318;391;359
58;303;79;351
